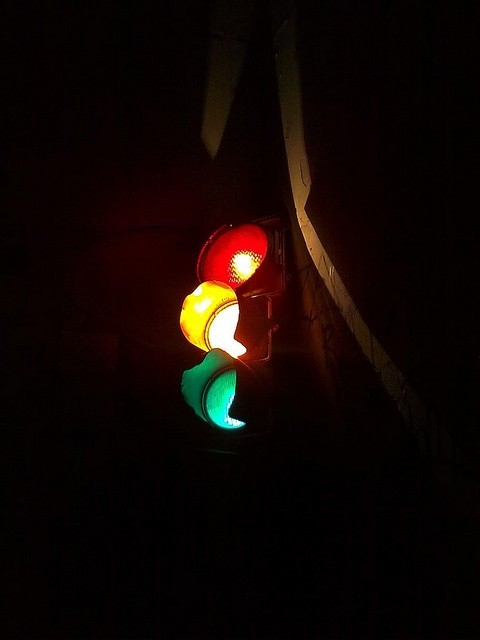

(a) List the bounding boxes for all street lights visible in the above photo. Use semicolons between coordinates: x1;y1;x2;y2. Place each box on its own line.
181;205;290;434
